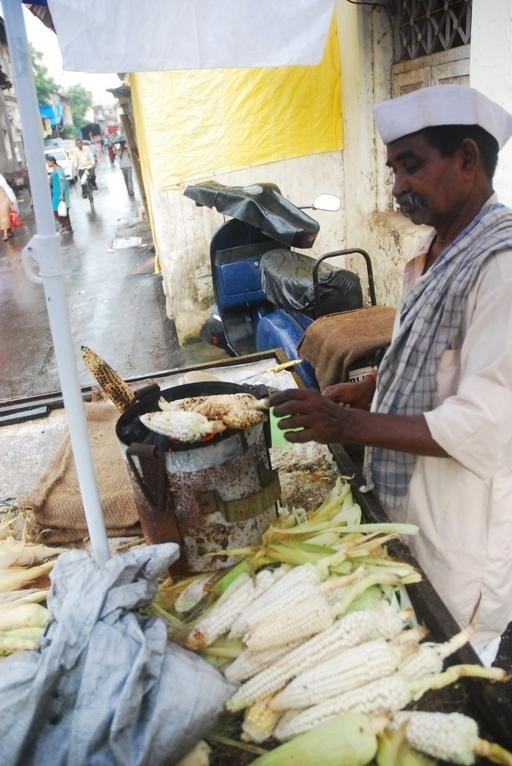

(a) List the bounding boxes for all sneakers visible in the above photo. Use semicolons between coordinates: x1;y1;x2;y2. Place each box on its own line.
60;226;73;235
3;231;13;241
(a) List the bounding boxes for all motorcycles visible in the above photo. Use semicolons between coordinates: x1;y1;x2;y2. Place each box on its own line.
183;177;399;405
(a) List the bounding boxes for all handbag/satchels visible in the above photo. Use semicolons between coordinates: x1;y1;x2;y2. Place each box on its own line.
9;210;23;226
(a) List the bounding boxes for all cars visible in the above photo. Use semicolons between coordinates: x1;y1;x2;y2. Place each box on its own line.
81;140;96;156
44;147;79;185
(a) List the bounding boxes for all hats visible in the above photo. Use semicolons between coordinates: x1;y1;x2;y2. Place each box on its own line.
374;85;512;151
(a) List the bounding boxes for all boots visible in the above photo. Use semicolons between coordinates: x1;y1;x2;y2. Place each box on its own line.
91;176;97;190
81;184;87;198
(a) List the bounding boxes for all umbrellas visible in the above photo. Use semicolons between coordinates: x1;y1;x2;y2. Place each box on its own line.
80;120;100;140
111;135;127;144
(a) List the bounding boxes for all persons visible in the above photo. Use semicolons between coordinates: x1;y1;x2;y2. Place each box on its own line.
258;81;512;668
116;141;134;194
45;155;73;234
0;174;19;240
89;131;118;164
72;137;97;198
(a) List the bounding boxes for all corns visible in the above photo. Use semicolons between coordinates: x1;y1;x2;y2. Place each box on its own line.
79;345;137;415
139;393;266;442
187;561;491;766
0;536;69;659
